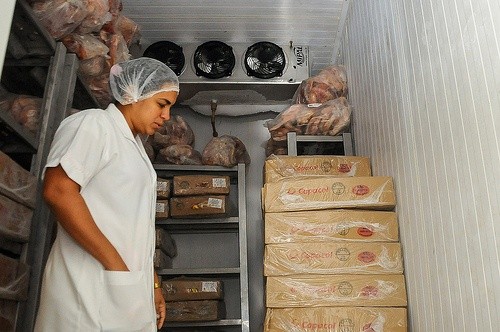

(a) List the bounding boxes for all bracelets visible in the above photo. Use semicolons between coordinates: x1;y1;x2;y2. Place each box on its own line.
153;281;162;290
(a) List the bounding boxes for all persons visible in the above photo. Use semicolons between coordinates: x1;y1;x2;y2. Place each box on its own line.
27;57;182;332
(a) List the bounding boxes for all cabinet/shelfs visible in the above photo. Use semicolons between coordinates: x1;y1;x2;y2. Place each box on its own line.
20;54;102;332
152;162;250;332
0;1;67;332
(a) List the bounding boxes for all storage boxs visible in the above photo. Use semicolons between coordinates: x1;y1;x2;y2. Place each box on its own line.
0;152;38;331
259;153;409;331
156;174;229;321
